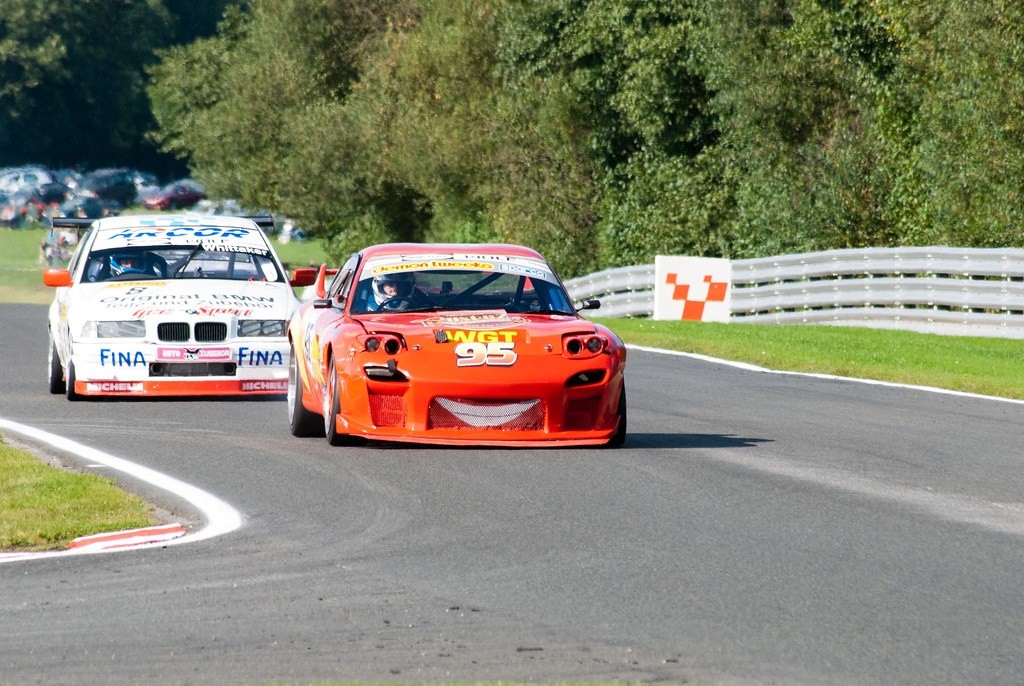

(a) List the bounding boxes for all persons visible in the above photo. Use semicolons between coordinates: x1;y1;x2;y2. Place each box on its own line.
366;270;430;314
108;248;150;281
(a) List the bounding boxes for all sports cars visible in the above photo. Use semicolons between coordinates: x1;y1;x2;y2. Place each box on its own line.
283;240;634;454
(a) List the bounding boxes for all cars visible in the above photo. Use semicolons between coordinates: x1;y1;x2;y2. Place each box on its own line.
1;165;309;240
42;209;317;406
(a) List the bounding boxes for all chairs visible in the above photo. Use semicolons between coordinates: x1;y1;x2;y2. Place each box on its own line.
141;252;169;279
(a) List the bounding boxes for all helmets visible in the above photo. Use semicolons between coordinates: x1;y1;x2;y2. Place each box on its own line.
372;272;417;310
109;249;149;276
